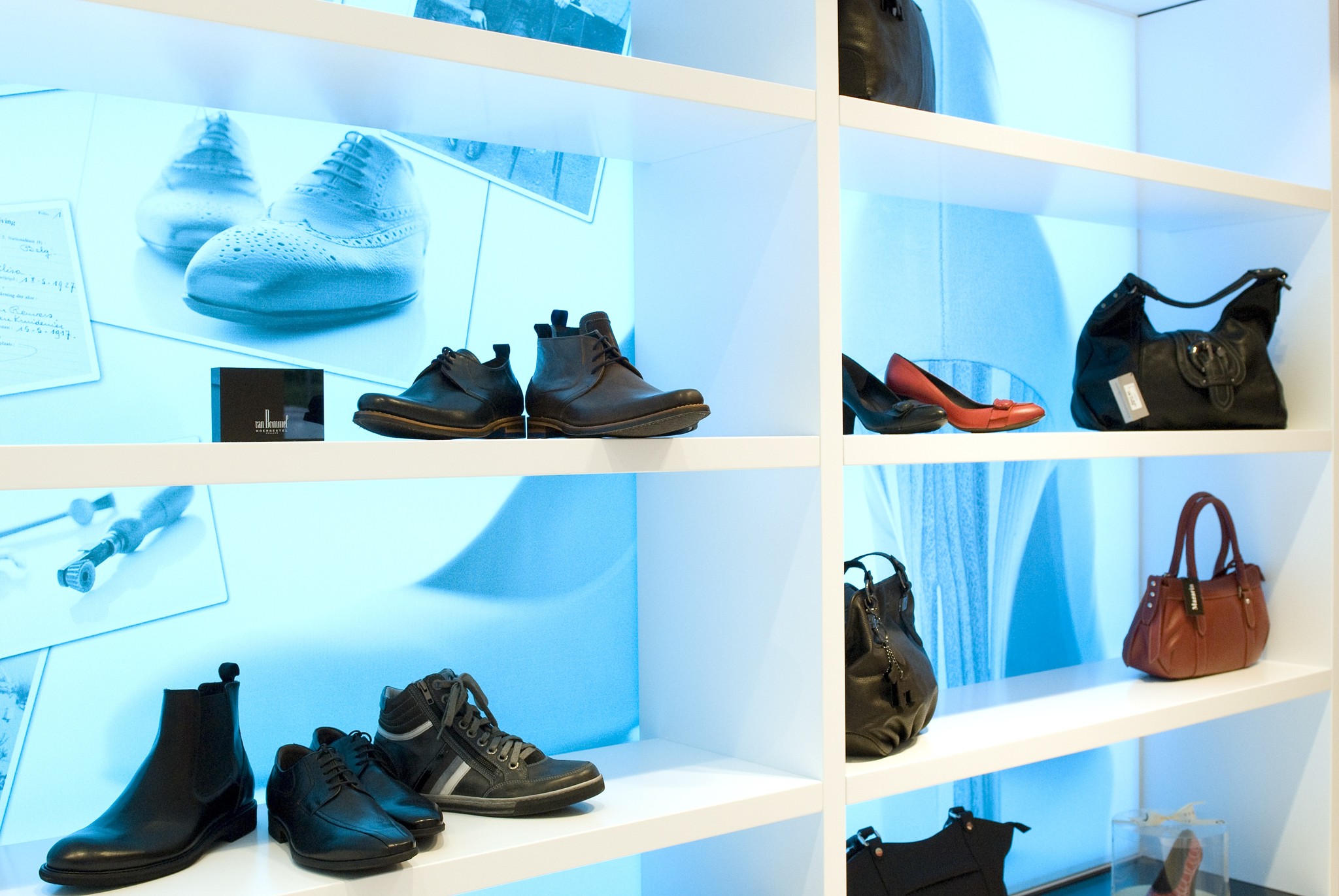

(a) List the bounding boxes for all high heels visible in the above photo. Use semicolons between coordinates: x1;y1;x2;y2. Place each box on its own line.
1146;830;1203;896
884;353;1045;432
842;352;947;434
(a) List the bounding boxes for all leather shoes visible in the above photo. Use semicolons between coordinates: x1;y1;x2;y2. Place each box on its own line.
528;310;711;439
266;726;446;871
350;343;526;439
37;663;257;887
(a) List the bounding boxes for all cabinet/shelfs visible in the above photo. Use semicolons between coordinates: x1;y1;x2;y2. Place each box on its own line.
1;0;1337;896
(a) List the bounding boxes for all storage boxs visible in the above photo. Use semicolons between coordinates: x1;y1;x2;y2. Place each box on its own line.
1108;807;1232;896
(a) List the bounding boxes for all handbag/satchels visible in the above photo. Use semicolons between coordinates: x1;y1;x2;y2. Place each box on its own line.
847;806;1030;896
839;1;936;112
842;551;939;758
1070;268;1291;431
1122;492;1270;680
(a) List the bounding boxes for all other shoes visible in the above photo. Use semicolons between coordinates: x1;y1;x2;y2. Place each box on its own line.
374;668;605;818
137;110;427;327
